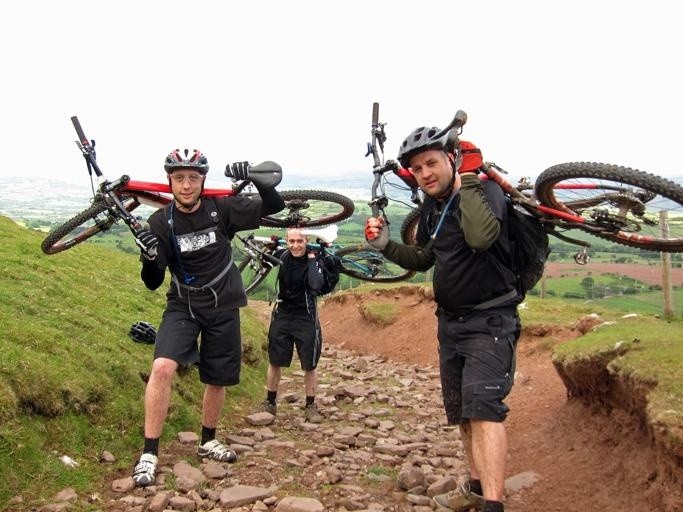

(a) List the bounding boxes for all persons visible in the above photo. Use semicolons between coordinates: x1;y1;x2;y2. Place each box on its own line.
365;126;520;512
132;148;285;485
251;228;324;423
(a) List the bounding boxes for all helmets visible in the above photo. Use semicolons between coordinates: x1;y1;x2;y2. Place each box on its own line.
127;321;158;344
164;148;209;175
397;126;459;169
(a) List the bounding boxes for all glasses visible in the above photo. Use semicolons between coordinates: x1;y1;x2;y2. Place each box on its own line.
170;174;203;181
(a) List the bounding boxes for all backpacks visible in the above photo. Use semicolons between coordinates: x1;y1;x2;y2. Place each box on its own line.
422;194;551;291
280;248;339;296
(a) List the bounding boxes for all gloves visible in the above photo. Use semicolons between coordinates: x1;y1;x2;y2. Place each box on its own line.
268;235;282;251
316;238;333;248
134;223;159;264
224;161;254;183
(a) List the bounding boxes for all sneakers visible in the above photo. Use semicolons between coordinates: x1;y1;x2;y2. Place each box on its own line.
248;400;276;415
197;439;237;462
133;451;158;486
305;403;321;422
433;481;484;511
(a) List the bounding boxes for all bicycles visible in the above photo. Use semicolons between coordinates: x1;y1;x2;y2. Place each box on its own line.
41;114;356;255
364;101;683;254
232;225;418;297
